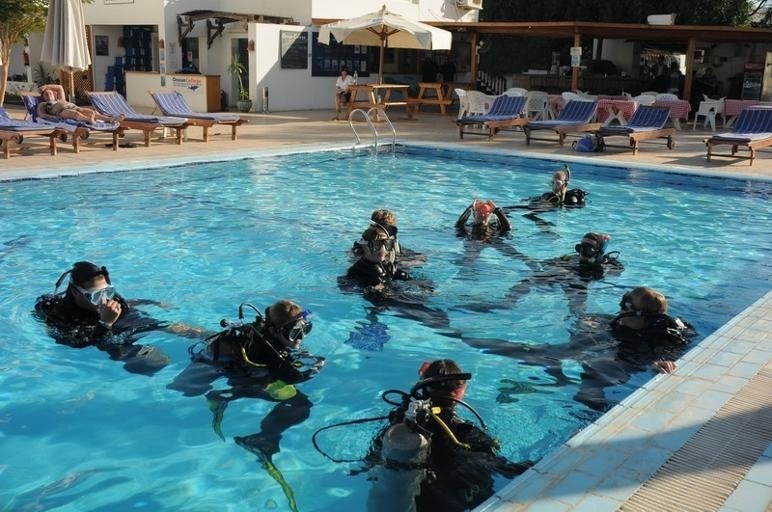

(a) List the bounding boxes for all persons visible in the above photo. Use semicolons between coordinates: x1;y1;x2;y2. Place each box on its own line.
497;170;586;231
332;65;355;121
165;299;327;512
336;208;463;339
448;232;625;319
639;55;684;98
36;84;126;124
34;260;220;379
422;56;458;98
462;286;700;414
454;197;543;273
701;67;718;96
311;358;543;512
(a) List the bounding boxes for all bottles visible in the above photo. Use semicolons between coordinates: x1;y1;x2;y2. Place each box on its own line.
353;70;358;85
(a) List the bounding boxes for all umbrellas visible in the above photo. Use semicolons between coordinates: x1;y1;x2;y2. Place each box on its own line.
317;5;453;85
39;0;93;105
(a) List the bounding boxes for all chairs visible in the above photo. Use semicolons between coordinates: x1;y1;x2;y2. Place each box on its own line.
524;91;548;121
703;94;727;130
454;88;468;119
502;87;528;130
465;90;495;130
562;92;579;103
656;94;681;130
693;102;719;131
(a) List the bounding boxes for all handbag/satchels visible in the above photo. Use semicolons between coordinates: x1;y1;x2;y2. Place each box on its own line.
575;135;604;152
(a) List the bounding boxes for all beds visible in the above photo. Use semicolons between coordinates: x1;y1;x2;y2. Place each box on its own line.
148;90;250;142
85;90;196;148
702;106;772;167
523;99;598;146
19;91;130;153
457;94;528;140
0;106;67;159
598;104;676;155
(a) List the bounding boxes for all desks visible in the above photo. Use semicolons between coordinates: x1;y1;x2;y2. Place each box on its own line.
345;84;412;120
598;99;640;127
724;99;760;130
653;100;691;130
414;82;474;114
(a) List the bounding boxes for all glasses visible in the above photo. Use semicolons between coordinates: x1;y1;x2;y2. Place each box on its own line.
341;68;348;72
